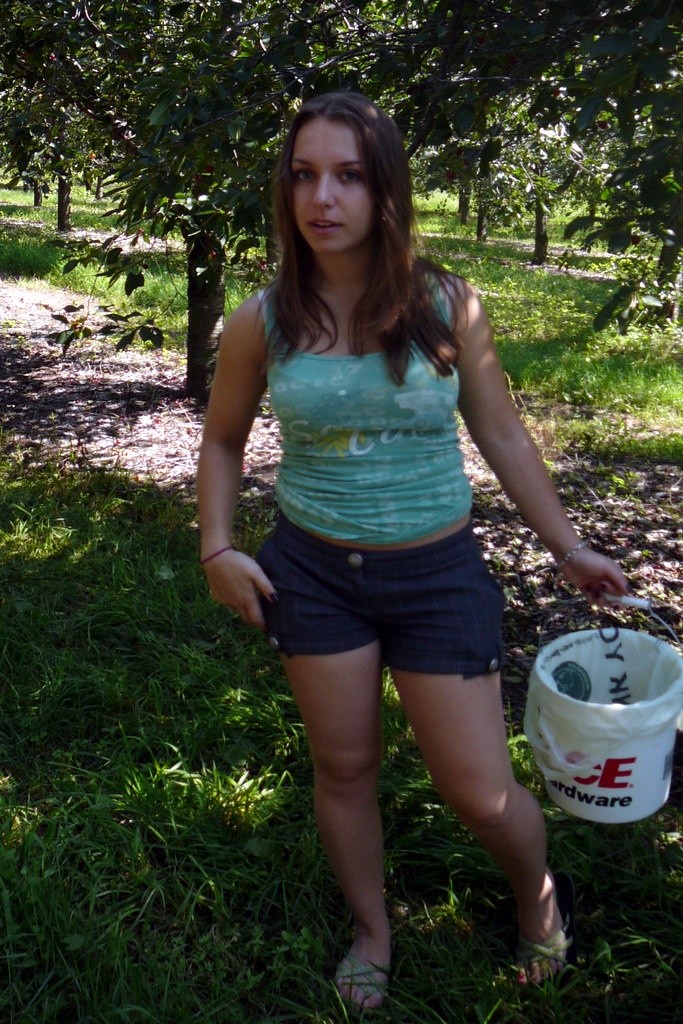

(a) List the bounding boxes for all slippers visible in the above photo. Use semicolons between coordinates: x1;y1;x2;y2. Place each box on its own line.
514;923;569;985
333;943;390;1009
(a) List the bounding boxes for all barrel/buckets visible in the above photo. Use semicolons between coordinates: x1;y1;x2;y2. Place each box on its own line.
524;592;683;824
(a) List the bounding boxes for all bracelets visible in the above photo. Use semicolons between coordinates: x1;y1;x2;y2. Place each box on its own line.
558;541;588;568
200;546;234;564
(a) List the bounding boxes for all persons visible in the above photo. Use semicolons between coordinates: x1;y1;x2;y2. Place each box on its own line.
198;93;631;1011
